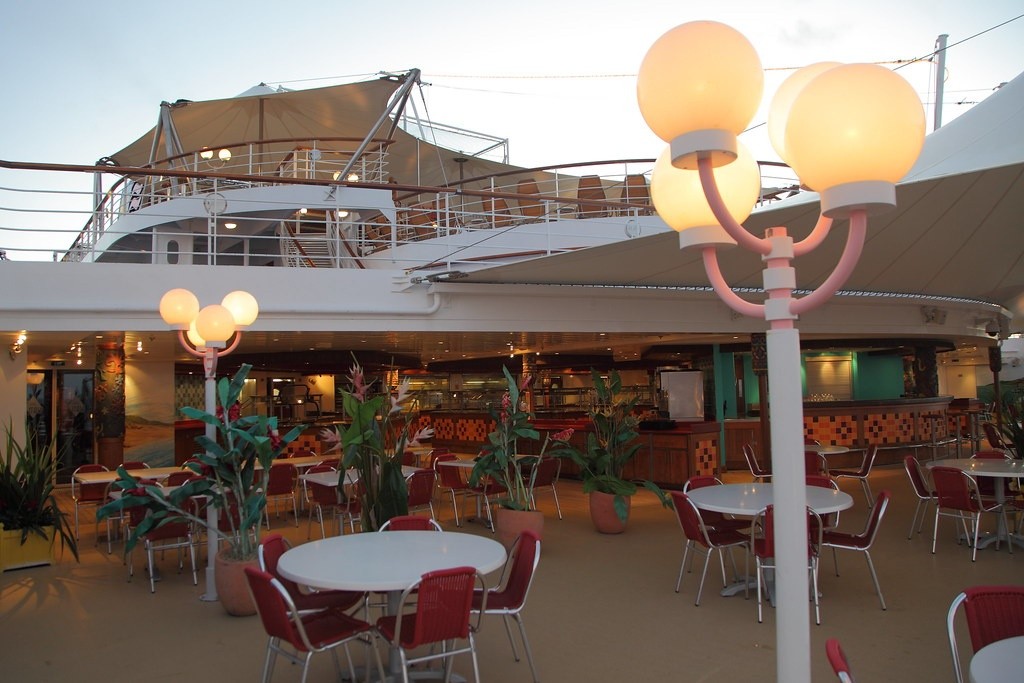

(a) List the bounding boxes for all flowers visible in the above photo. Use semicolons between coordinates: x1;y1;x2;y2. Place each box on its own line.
318;350;438;530
97;361;311;565
550;363;676;526
469;363;574;510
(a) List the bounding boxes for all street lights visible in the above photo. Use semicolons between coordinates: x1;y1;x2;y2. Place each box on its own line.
157;286;261;608
632;19;927;683
199;146;232;265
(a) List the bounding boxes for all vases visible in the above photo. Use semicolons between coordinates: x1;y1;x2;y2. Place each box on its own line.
215;542;265;618
497;506;543;558
590;490;630;534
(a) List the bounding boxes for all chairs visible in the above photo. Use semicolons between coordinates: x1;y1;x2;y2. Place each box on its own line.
365;175;649;250
71;422;1024;682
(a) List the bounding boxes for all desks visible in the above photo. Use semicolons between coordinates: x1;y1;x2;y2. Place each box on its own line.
108;485;263;582
298;463;428;536
277;531;507;683
687;483;856;608
968;636;1024;683
926;459;1024;551
805;445;850;454
437;454;540;529
76;446;436;541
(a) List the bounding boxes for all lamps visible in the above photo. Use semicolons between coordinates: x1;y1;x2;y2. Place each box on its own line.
9;339;24;360
922;304;937;321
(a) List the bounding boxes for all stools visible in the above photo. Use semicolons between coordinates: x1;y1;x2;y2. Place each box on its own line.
922;408;986;462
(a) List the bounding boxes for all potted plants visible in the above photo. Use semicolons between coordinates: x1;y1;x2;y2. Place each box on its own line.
976;381;1024;534
0;412;81;573
